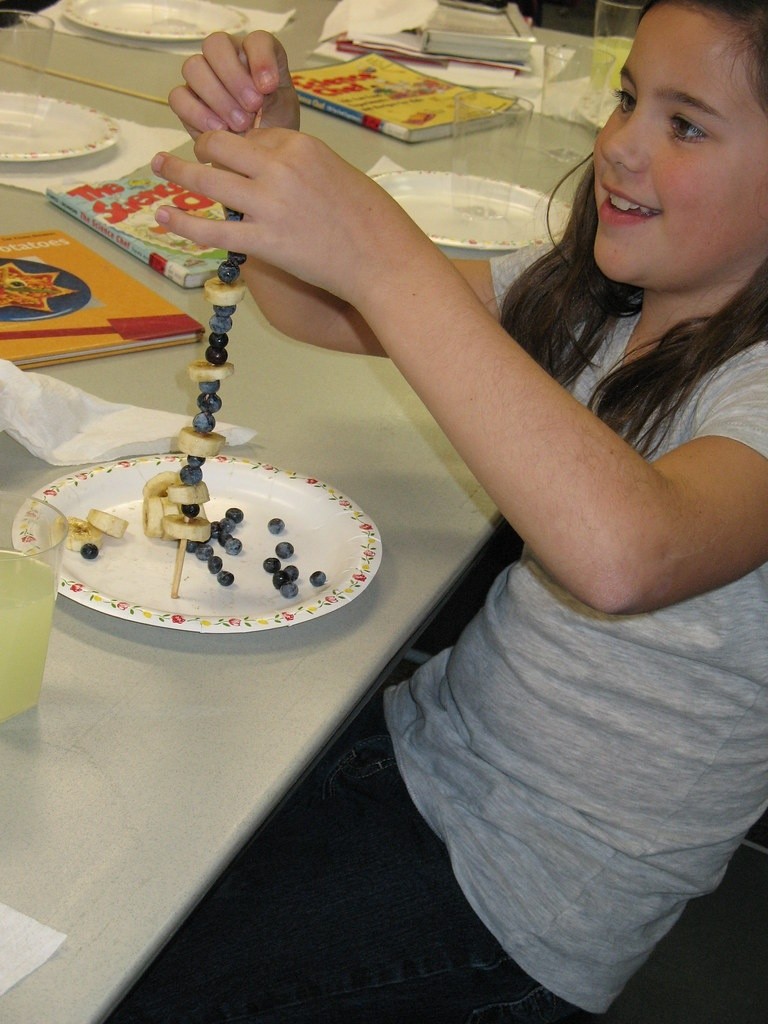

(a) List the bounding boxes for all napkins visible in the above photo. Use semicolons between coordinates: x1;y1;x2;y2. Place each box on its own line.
0;360;259;468
1;0;644;261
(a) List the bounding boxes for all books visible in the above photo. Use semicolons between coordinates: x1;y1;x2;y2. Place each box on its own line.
0;228;205;378
279;51;531;144
44;154;266;292
323;1;536;76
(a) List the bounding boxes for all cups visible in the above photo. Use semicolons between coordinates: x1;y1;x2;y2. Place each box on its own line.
449;88;535;219
541;42;616;164
0;10;55;139
0;491;71;725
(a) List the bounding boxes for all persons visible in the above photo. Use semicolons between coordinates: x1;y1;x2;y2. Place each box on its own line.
104;0;768;1024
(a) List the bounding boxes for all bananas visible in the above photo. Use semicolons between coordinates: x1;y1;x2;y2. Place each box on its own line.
54;470;212;553
205;277;247;306
189;360;234;382
178;426;225;456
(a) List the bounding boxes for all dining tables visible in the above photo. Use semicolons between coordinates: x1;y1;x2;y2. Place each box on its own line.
0;61;583;1021
0;0;630;209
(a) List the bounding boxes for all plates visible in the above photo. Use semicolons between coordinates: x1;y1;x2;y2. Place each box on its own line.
0;91;120;163
11;452;382;635
366;174;574;255
60;0;250;43
